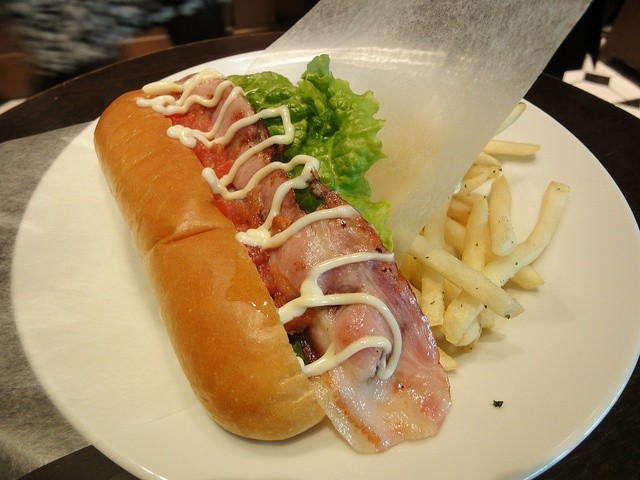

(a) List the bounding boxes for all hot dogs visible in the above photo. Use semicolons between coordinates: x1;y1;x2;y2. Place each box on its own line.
93;68;451;456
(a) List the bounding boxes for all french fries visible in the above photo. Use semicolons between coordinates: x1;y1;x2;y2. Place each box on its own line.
397;105;570;372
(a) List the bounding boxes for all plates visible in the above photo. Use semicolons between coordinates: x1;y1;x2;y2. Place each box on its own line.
10;49;640;480
